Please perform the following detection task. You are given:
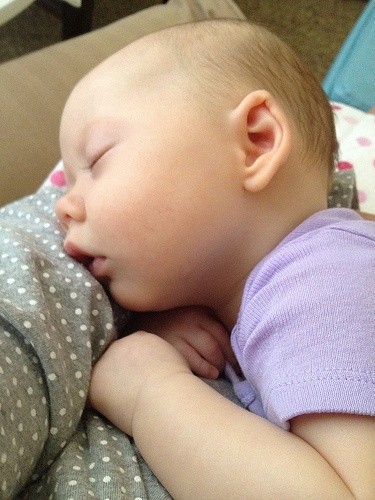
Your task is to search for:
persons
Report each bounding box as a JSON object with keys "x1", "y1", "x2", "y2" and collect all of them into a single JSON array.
[{"x1": 51, "y1": 17, "x2": 375, "y2": 500}]
